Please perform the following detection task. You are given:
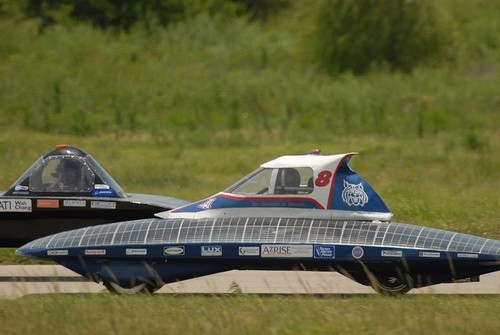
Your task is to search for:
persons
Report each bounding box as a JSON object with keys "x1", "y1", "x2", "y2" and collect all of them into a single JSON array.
[
  {"x1": 272, "y1": 169, "x2": 303, "y2": 198},
  {"x1": 48, "y1": 158, "x2": 84, "y2": 191}
]
